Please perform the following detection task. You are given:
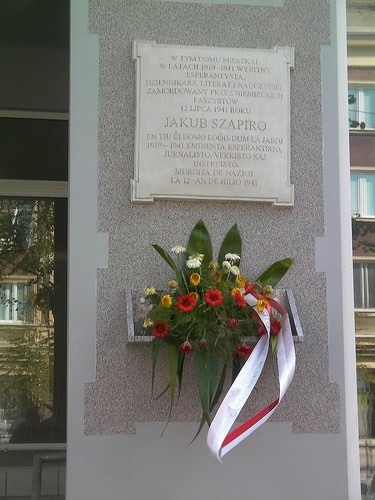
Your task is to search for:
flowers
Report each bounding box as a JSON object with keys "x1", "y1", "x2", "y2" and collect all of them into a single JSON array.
[{"x1": 145, "y1": 220, "x2": 299, "y2": 460}]
